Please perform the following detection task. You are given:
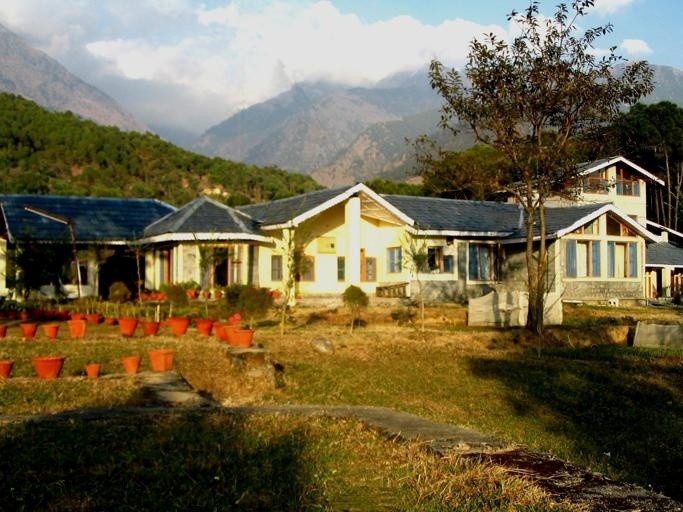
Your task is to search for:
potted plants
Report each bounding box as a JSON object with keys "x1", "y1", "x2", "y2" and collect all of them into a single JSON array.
[{"x1": 0, "y1": 279, "x2": 281, "y2": 379}]
